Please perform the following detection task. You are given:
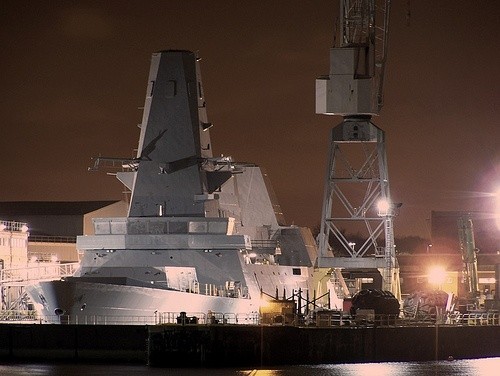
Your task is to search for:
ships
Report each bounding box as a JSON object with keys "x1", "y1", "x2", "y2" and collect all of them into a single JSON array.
[{"x1": 22, "y1": 1, "x2": 406, "y2": 325}]
